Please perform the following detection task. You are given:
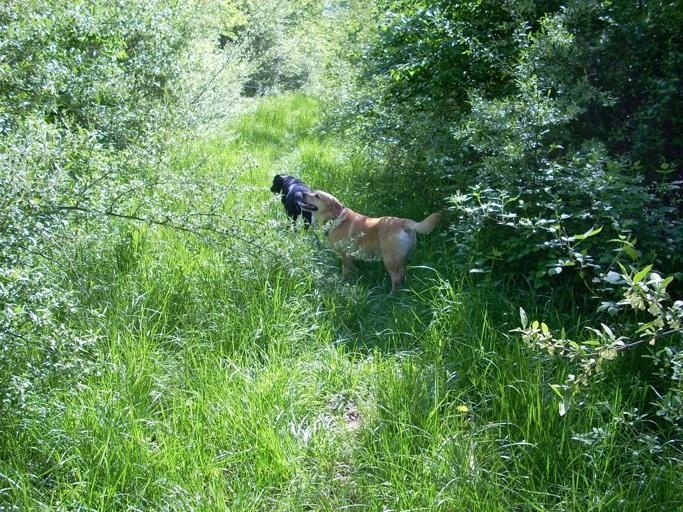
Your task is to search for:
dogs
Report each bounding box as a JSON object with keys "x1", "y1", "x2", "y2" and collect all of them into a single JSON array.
[
  {"x1": 300, "y1": 189, "x2": 441, "y2": 298},
  {"x1": 270, "y1": 174, "x2": 313, "y2": 231}
]
